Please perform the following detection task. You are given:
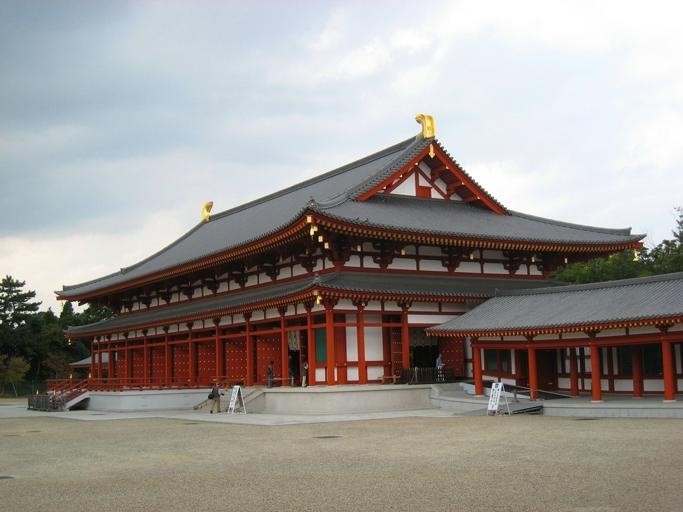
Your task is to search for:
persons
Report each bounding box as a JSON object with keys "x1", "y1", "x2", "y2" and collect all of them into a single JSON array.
[
  {"x1": 301, "y1": 354, "x2": 309, "y2": 387},
  {"x1": 435, "y1": 353, "x2": 446, "y2": 382},
  {"x1": 210, "y1": 378, "x2": 224, "y2": 413},
  {"x1": 267, "y1": 360, "x2": 274, "y2": 386}
]
